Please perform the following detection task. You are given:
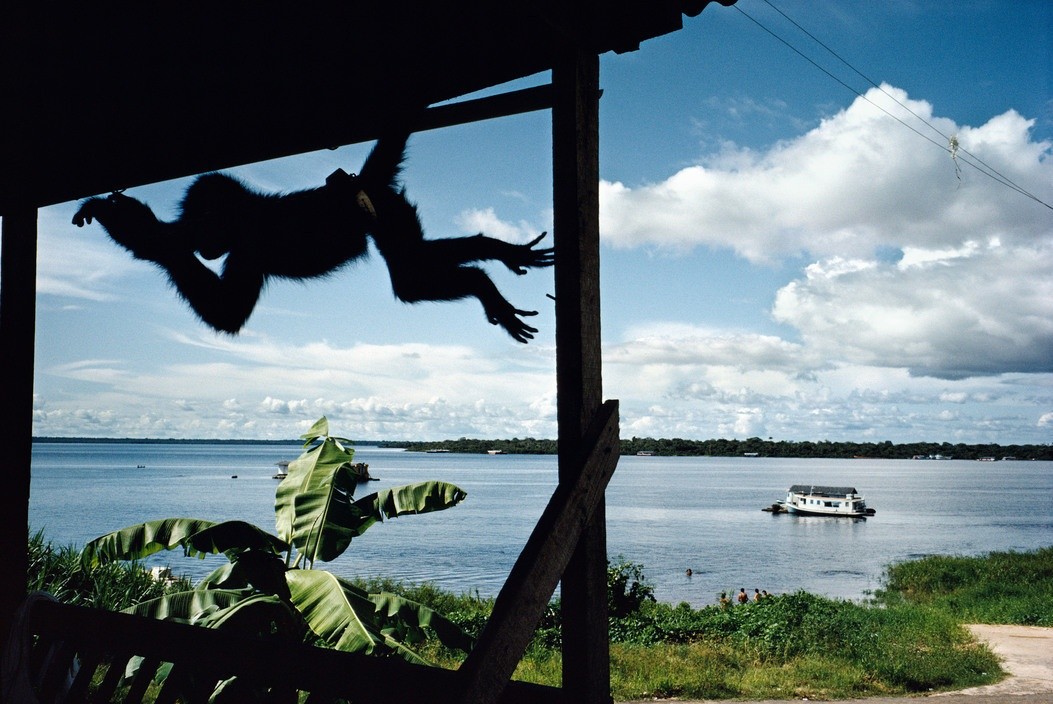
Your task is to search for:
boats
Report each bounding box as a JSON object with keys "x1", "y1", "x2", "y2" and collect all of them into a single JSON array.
[
  {"x1": 744, "y1": 452, "x2": 759, "y2": 457},
  {"x1": 911, "y1": 454, "x2": 954, "y2": 460},
  {"x1": 977, "y1": 456, "x2": 996, "y2": 462},
  {"x1": 427, "y1": 448, "x2": 450, "y2": 453},
  {"x1": 762, "y1": 484, "x2": 876, "y2": 517},
  {"x1": 487, "y1": 449, "x2": 504, "y2": 454},
  {"x1": 1002, "y1": 456, "x2": 1017, "y2": 461},
  {"x1": 636, "y1": 450, "x2": 656, "y2": 456},
  {"x1": 272, "y1": 461, "x2": 371, "y2": 483}
]
